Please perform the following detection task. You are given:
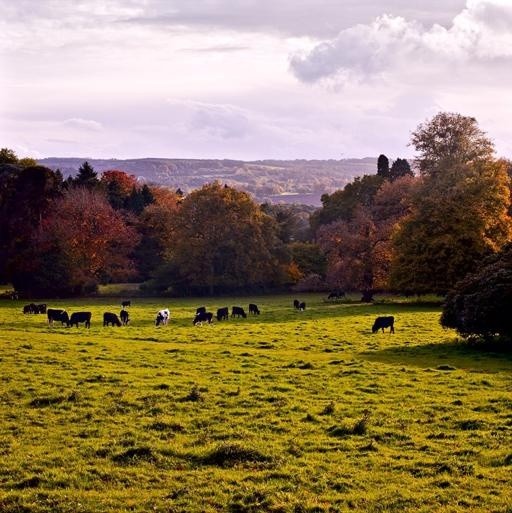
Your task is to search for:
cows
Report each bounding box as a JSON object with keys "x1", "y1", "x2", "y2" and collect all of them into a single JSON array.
[
  {"x1": 101, "y1": 300, "x2": 132, "y2": 328},
  {"x1": 371, "y1": 315, "x2": 395, "y2": 334},
  {"x1": 328, "y1": 290, "x2": 348, "y2": 300},
  {"x1": 293, "y1": 299, "x2": 307, "y2": 311},
  {"x1": 154, "y1": 307, "x2": 170, "y2": 326},
  {"x1": 191, "y1": 303, "x2": 261, "y2": 326},
  {"x1": 22, "y1": 302, "x2": 93, "y2": 329}
]
